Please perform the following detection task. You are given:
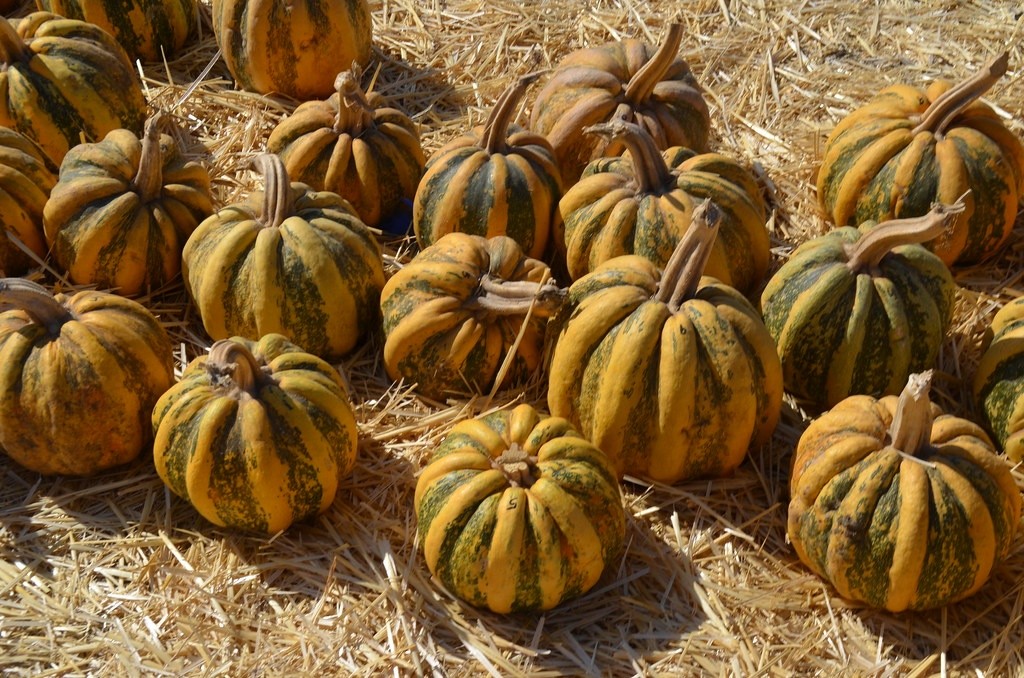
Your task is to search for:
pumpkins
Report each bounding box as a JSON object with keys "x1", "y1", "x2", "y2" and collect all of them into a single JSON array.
[{"x1": 0, "y1": 0, "x2": 1024, "y2": 614}]
